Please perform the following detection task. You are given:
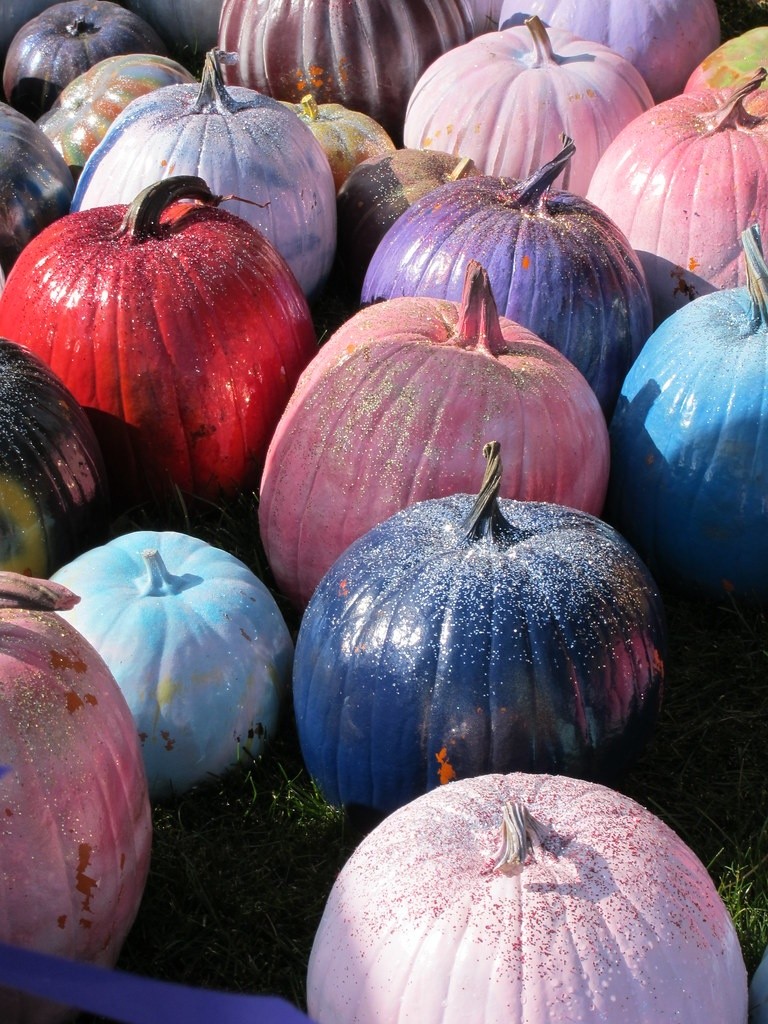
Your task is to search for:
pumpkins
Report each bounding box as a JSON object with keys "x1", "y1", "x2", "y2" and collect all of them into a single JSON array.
[{"x1": 0, "y1": 0, "x2": 768, "y2": 1024}]
